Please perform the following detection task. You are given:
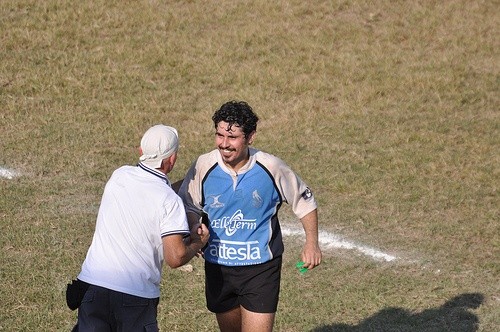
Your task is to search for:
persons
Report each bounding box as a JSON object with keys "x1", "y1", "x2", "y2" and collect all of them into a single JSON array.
[
  {"x1": 74, "y1": 124, "x2": 210, "y2": 332},
  {"x1": 178, "y1": 100, "x2": 322, "y2": 332}
]
